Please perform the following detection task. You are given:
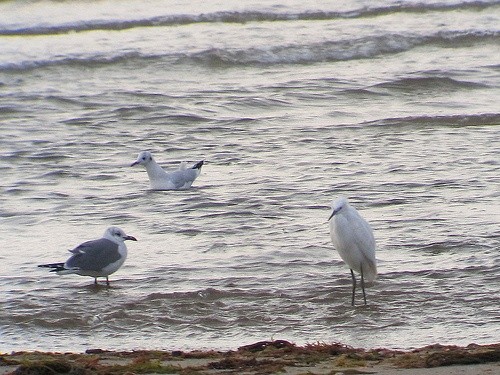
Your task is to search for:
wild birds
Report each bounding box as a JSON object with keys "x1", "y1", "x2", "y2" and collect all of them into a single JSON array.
[
  {"x1": 130, "y1": 151, "x2": 204, "y2": 190},
  {"x1": 36, "y1": 226, "x2": 137, "y2": 285},
  {"x1": 327, "y1": 197, "x2": 377, "y2": 308}
]
